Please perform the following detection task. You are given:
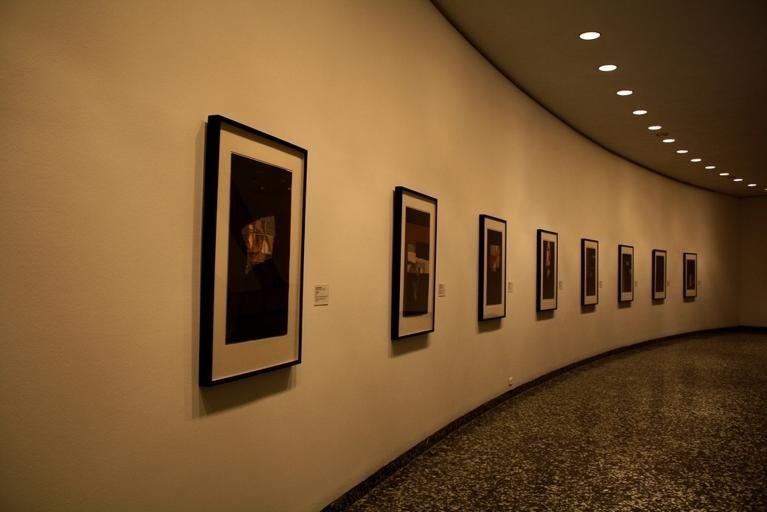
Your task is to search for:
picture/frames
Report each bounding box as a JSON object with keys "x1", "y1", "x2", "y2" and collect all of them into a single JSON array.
[
  {"x1": 200, "y1": 114, "x2": 307, "y2": 388},
  {"x1": 392, "y1": 186, "x2": 437, "y2": 340},
  {"x1": 479, "y1": 215, "x2": 506, "y2": 321},
  {"x1": 537, "y1": 230, "x2": 697, "y2": 311}
]
